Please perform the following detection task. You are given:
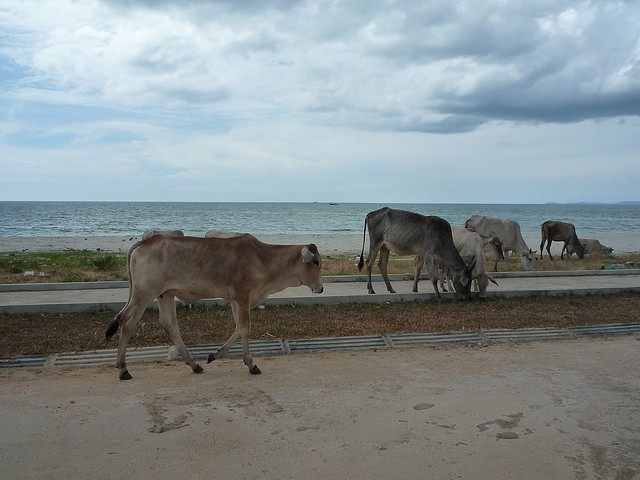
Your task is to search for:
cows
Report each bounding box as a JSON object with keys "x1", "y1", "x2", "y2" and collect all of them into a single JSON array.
[
  {"x1": 104, "y1": 234, "x2": 325, "y2": 381},
  {"x1": 563, "y1": 236, "x2": 615, "y2": 260},
  {"x1": 468, "y1": 229, "x2": 505, "y2": 267},
  {"x1": 464, "y1": 214, "x2": 539, "y2": 271},
  {"x1": 436, "y1": 224, "x2": 499, "y2": 300},
  {"x1": 356, "y1": 205, "x2": 482, "y2": 304},
  {"x1": 539, "y1": 220, "x2": 586, "y2": 261}
]
